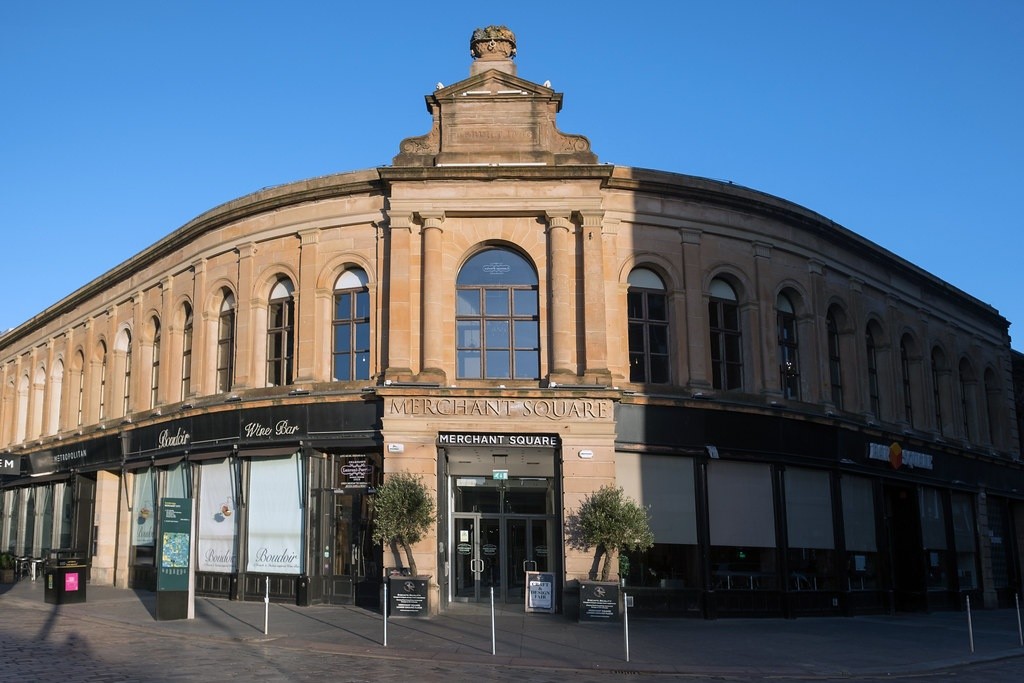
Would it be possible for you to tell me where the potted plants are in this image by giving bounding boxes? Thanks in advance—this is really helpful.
[362,471,433,620]
[0,552,15,583]
[564,488,655,626]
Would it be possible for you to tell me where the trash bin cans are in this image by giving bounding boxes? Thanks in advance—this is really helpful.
[43,548,87,605]
